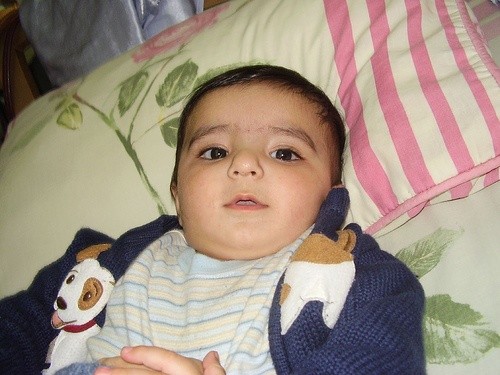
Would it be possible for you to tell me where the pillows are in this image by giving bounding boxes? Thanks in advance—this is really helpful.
[0,0,500,300]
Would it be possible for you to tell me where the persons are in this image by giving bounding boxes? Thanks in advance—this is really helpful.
[1,66,426,375]
